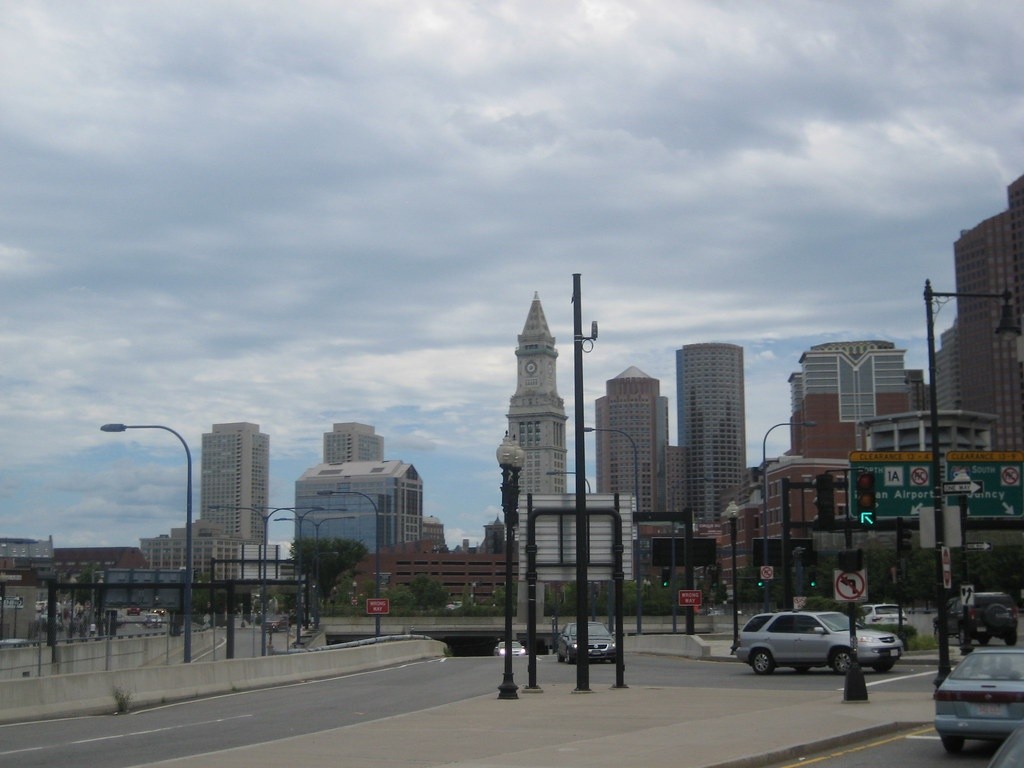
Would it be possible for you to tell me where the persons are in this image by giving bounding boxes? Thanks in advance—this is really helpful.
[89,622,96,637]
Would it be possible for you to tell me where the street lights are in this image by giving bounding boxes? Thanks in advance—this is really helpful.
[923,278,1024,689]
[673,476,712,634]
[13,596,19,638]
[100,423,192,662]
[545,427,641,633]
[497,431,525,700]
[206,488,380,656]
[763,421,817,613]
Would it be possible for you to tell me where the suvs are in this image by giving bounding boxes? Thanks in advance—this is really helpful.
[731,593,1017,675]
[262,613,290,632]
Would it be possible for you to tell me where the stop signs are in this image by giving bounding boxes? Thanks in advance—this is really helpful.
[941,547,952,589]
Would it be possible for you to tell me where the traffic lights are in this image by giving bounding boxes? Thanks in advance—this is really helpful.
[757,576,763,587]
[856,472,876,527]
[662,569,670,588]
[810,572,816,587]
[897,517,912,551]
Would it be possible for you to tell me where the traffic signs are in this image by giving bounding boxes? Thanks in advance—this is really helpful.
[849,450,1024,519]
[967,542,991,550]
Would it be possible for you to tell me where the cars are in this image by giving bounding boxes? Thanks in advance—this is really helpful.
[494,622,616,664]
[934,643,1024,768]
[126,607,166,628]
